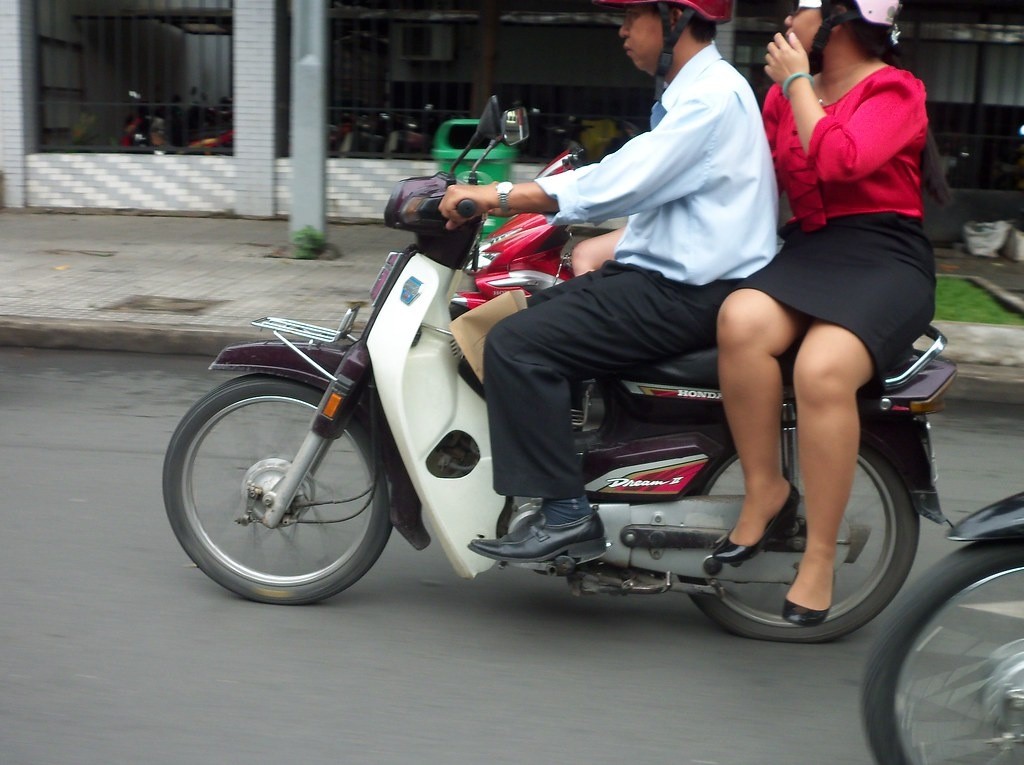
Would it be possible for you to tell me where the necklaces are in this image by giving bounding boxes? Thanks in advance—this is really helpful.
[818,95,824,105]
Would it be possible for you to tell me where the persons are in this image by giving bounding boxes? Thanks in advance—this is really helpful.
[711,0,944,626]
[439,0,783,569]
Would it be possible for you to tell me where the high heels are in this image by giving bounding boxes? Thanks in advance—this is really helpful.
[781,571,835,628]
[713,482,800,563]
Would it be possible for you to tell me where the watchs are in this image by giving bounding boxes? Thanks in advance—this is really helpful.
[497,181,514,216]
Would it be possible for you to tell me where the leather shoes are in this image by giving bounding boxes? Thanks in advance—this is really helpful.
[467,511,606,563]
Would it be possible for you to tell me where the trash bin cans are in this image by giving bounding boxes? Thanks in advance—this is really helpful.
[429,118,521,238]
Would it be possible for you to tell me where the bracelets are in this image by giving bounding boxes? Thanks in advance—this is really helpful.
[783,72,815,99]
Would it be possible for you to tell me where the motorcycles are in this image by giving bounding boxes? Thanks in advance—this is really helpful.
[860,492,1023,765]
[164,94,958,646]
[121,90,434,160]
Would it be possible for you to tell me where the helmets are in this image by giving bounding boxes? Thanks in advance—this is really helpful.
[597,0,733,25]
[787,0,900,27]
